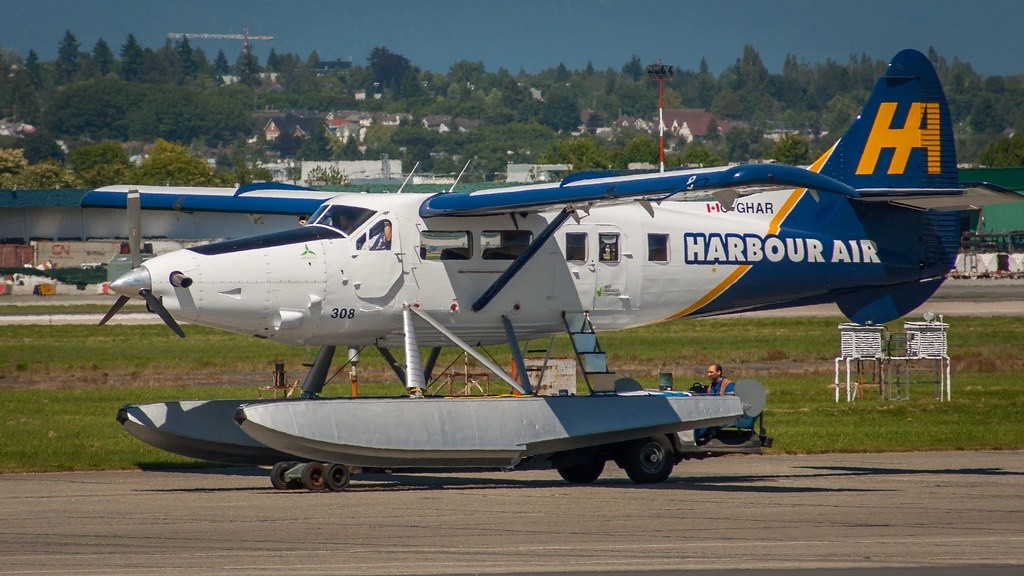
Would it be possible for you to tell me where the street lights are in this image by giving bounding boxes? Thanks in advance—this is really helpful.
[645,57,673,171]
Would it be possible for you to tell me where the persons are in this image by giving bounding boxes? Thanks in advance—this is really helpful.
[362,220,391,250]
[339,214,364,243]
[694,364,734,436]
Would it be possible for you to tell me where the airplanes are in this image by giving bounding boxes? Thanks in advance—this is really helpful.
[76,48,981,488]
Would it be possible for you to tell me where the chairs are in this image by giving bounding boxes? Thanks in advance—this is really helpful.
[418,246,585,260]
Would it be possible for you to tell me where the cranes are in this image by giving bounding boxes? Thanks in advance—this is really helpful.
[167,26,276,54]
